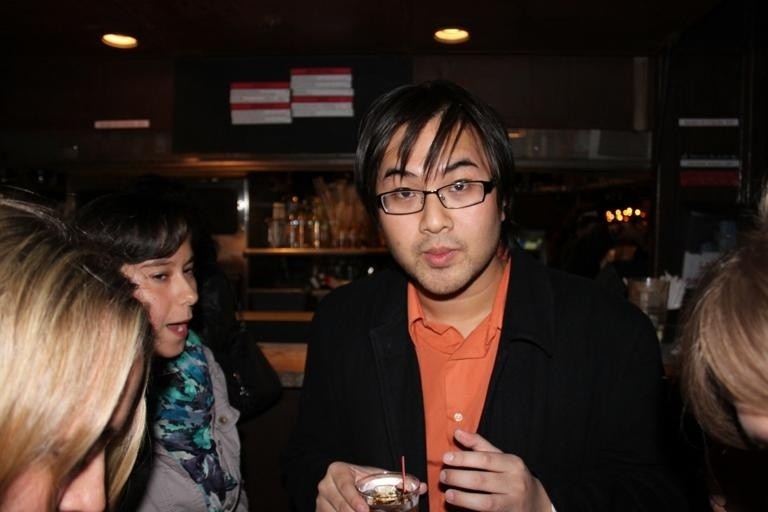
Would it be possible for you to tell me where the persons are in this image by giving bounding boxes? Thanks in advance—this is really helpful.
[169,193,283,420]
[0,183,154,511]
[271,75,717,511]
[664,233,767,511]
[64,179,255,512]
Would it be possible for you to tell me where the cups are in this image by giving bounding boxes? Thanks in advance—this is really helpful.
[355,471,422,512]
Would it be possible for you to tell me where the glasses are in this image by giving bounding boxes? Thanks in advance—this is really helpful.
[373,176,500,217]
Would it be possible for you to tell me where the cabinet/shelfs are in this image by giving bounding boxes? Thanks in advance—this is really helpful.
[83,151,668,392]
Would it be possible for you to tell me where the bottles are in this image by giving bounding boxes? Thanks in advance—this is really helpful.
[284,195,327,248]
